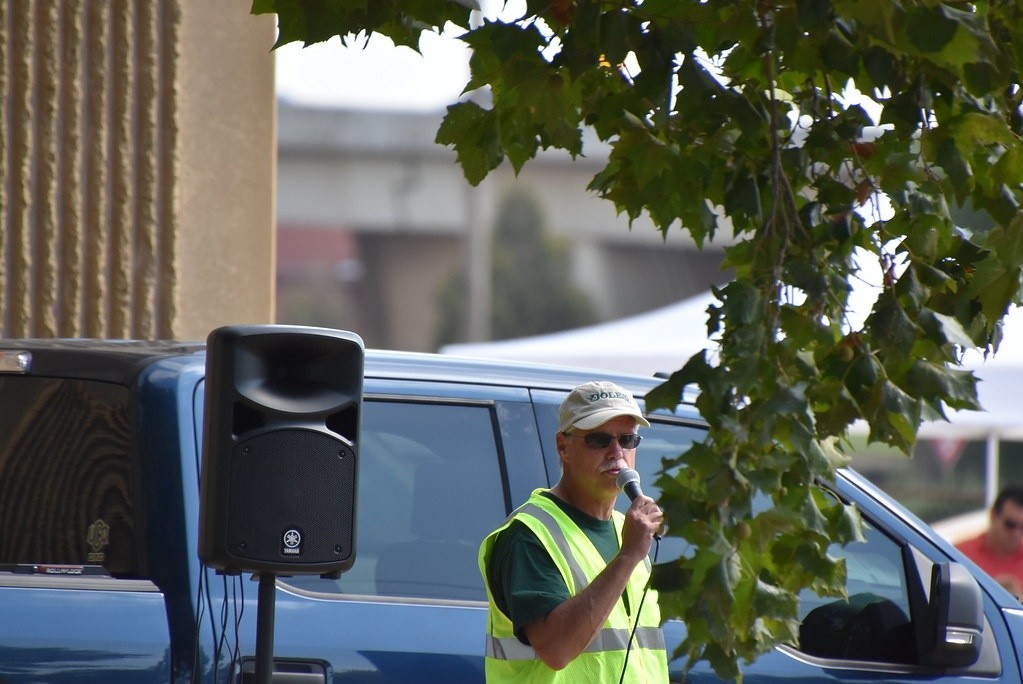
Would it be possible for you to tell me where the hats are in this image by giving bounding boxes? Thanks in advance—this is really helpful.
[555,380,651,433]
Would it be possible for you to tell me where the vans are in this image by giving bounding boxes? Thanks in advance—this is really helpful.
[0,325,1021,684]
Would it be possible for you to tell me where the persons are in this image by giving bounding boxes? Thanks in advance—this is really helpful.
[953,486,1023,602]
[477,380,671,684]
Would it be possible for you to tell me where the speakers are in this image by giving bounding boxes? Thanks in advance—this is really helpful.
[197,324,366,580]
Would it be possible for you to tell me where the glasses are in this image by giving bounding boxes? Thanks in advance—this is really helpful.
[568,431,643,450]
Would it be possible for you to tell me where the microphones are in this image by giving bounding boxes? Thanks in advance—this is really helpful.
[615,467,662,542]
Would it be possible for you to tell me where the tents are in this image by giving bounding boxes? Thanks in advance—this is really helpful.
[438,179,1023,509]
